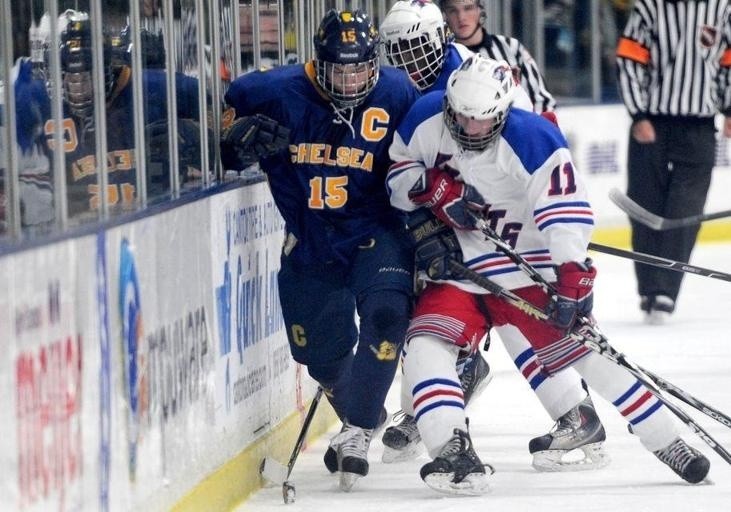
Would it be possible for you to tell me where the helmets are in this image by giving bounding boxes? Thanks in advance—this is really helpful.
[442,54,512,150]
[313,9,379,106]
[57,19,110,115]
[377,0,450,91]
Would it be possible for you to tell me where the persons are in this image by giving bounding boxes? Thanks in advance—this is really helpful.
[383,53,718,494]
[219,7,429,493]
[454,0,558,122]
[616,0,731,312]
[378,0,608,473]
[2,0,299,253]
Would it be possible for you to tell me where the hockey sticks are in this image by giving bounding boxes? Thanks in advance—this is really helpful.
[609,188,730,231]
[283,384,324,504]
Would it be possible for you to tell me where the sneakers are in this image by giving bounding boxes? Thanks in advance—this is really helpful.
[324,406,420,477]
[419,430,484,482]
[654,437,710,484]
[529,395,605,453]
[454,348,489,409]
[640,295,675,313]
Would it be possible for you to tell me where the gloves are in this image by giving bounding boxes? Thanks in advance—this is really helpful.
[146,117,216,173]
[224,114,291,170]
[543,258,596,329]
[403,168,484,279]
